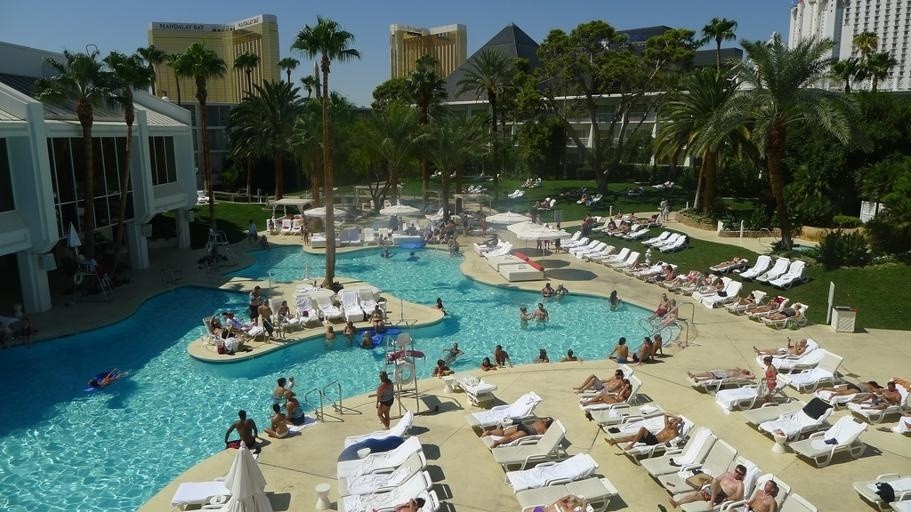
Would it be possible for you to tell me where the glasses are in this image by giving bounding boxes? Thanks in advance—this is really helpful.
[736,470,744,475]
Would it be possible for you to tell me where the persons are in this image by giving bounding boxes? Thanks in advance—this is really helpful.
[264,376,304,439]
[205,279,383,347]
[0,317,35,346]
[520,283,568,329]
[465,185,470,195]
[668,464,747,508]
[429,207,468,256]
[482,416,551,448]
[648,256,802,329]
[604,416,683,453]
[609,290,621,310]
[577,189,669,238]
[728,480,778,512]
[433,343,577,380]
[383,246,388,258]
[249,219,274,248]
[571,334,663,406]
[528,493,587,512]
[816,380,910,415]
[375,370,394,431]
[91,367,130,389]
[373,498,424,511]
[686,335,808,395]
[534,199,550,207]
[225,410,258,449]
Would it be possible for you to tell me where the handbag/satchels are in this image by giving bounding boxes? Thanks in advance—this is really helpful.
[718,291,727,296]
[686,473,713,490]
[876,482,894,501]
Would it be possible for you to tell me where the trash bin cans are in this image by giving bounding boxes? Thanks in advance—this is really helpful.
[831,306,857,333]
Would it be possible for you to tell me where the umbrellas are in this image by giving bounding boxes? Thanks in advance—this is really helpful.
[379,202,420,229]
[518,227,571,272]
[225,441,272,511]
[303,205,347,237]
[507,221,545,256]
[67,221,84,273]
[485,211,532,243]
